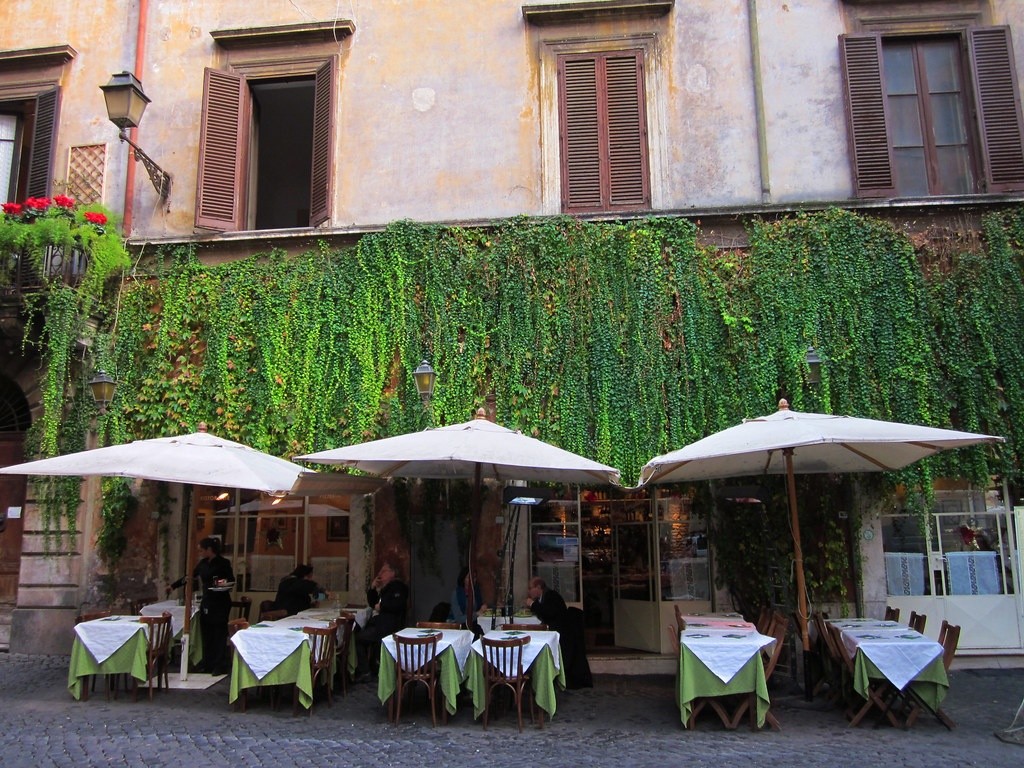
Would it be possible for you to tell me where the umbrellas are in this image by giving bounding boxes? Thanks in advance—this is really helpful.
[638,397,1007,704]
[0,421,319,682]
[290,407,623,633]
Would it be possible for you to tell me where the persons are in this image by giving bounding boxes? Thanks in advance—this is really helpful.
[524,577,593,696]
[162,538,235,677]
[446,566,496,642]
[353,559,409,684]
[268,563,337,616]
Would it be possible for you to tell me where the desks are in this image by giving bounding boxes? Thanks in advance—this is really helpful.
[67,615,158,702]
[464,629,567,730]
[840,627,958,732]
[675,629,778,730]
[476,612,543,638]
[225,621,331,716]
[825,617,902,634]
[682,612,756,631]
[299,608,371,686]
[140,599,203,667]
[377,626,475,716]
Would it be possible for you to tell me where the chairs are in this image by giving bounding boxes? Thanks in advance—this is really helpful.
[74,602,962,736]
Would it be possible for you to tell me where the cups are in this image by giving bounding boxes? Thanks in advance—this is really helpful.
[483,609,493,616]
[908,627,914,636]
[496,609,502,616]
[212,576,218,588]
[524,608,531,616]
[880,620,885,627]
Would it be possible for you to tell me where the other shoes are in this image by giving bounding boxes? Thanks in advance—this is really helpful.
[192,659,212,672]
[213,666,226,676]
[354,672,371,685]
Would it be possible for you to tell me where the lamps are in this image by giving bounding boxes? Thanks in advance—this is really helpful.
[100,71,174,199]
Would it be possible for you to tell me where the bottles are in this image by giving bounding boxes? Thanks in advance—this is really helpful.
[531,483,707,575]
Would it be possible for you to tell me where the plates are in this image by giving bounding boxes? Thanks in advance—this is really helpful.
[208,581,236,592]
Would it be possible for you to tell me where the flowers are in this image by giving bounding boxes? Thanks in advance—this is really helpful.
[0,195,108,233]
